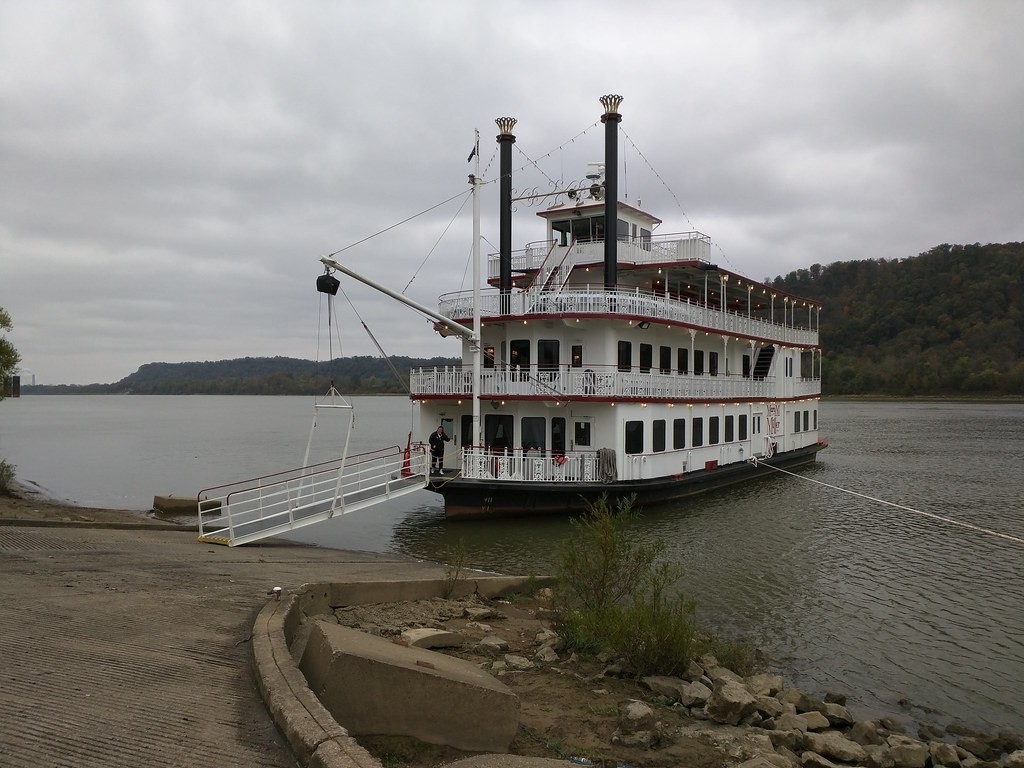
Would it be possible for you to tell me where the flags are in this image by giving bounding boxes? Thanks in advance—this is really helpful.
[467,140,479,162]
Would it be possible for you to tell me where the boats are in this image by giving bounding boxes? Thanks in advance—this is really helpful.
[317,94,831,523]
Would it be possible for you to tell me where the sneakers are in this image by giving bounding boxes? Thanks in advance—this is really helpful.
[439,469,444,474]
[431,467,435,473]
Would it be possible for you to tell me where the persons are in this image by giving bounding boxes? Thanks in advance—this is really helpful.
[429,425,450,475]
